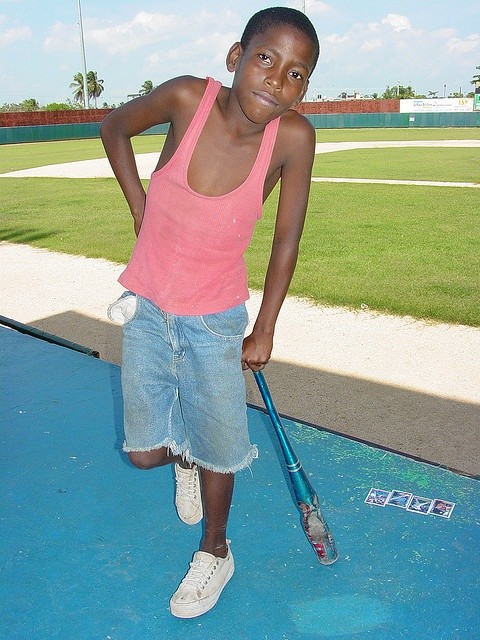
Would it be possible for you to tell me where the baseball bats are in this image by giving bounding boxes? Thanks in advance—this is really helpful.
[252,370,339,565]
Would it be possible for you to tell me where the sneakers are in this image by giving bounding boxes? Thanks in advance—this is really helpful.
[170,539,235,619]
[174,462,204,526]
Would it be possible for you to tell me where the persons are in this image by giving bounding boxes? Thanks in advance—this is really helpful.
[100,7,320,619]
